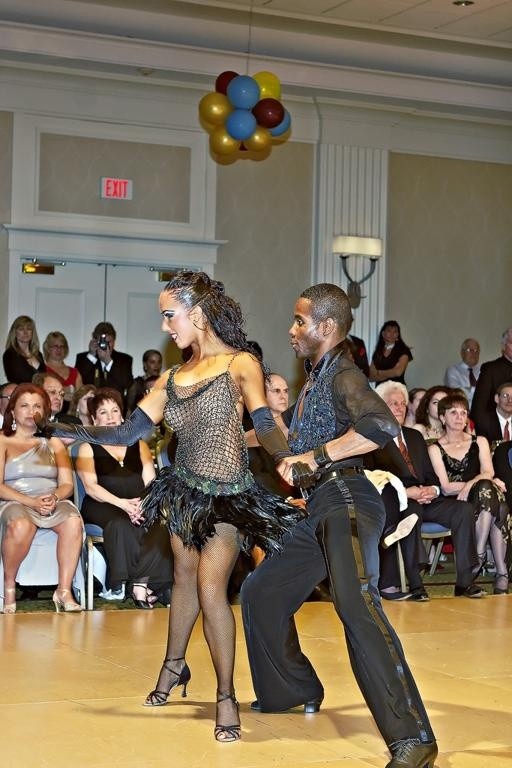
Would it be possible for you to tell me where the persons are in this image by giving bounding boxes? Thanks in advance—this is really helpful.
[0,316,173,616]
[33,266,318,742]
[360,319,512,602]
[238,281,440,766]
[239,374,307,510]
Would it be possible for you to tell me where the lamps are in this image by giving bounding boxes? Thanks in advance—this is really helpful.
[329,232,385,309]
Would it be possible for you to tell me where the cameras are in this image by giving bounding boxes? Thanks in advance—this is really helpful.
[97,334,110,351]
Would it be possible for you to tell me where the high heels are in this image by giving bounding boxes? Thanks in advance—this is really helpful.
[144,657,191,707]
[2,588,17,614]
[494,570,509,595]
[53,588,82,612]
[120,581,151,609]
[214,689,241,743]
[472,551,487,581]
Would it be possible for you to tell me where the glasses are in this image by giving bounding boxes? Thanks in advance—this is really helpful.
[465,348,478,352]
[503,393,508,399]
[47,389,65,397]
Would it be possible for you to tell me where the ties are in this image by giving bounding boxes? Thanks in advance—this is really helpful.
[398,434,414,478]
[469,368,476,386]
[504,422,509,441]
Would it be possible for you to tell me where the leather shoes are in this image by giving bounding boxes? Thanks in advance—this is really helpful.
[385,738,438,768]
[380,513,420,550]
[250,688,324,712]
[379,584,487,600]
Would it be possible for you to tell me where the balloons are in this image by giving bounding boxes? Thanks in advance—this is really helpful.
[194,65,297,167]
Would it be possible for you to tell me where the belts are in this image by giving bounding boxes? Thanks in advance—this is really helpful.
[315,466,365,488]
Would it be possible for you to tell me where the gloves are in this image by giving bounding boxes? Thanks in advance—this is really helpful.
[33,405,154,446]
[249,407,320,489]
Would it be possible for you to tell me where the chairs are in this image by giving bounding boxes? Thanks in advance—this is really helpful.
[1,436,81,612]
[71,442,109,616]
[419,516,455,578]
